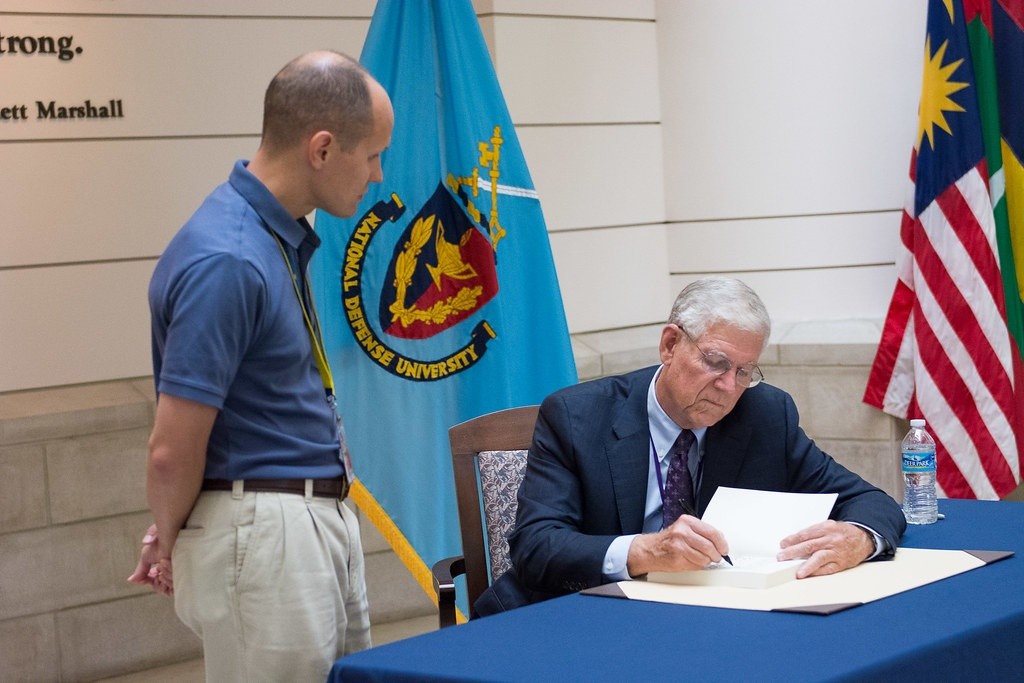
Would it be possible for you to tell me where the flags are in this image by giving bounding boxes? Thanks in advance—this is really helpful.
[860,0,1023,501]
[308,0,579,624]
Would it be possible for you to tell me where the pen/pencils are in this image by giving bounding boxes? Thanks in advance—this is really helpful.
[678,498,732,565]
[905,514,945,520]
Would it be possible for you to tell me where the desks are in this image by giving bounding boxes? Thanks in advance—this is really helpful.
[326,500,1024,683]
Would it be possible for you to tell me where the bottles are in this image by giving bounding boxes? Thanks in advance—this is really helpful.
[900,419,939,525]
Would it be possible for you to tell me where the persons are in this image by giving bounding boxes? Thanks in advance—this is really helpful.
[464,276,907,624]
[125,50,396,683]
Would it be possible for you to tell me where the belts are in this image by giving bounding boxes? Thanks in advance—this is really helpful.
[201,475,351,502]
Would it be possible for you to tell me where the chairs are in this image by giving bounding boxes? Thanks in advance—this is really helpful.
[432,404,542,627]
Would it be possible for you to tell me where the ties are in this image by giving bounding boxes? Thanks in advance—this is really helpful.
[663,429,698,531]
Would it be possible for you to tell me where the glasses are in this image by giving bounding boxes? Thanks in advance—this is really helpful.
[678,324,764,389]
